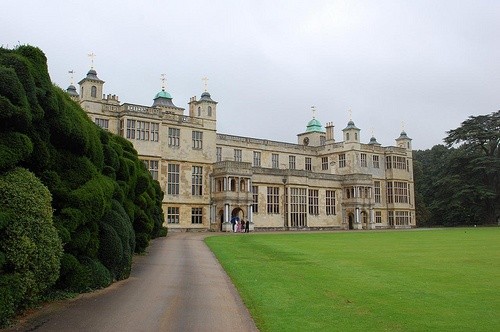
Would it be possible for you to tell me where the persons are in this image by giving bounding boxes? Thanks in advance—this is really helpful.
[245,219,250,233]
[240,219,245,233]
[230,217,241,233]
